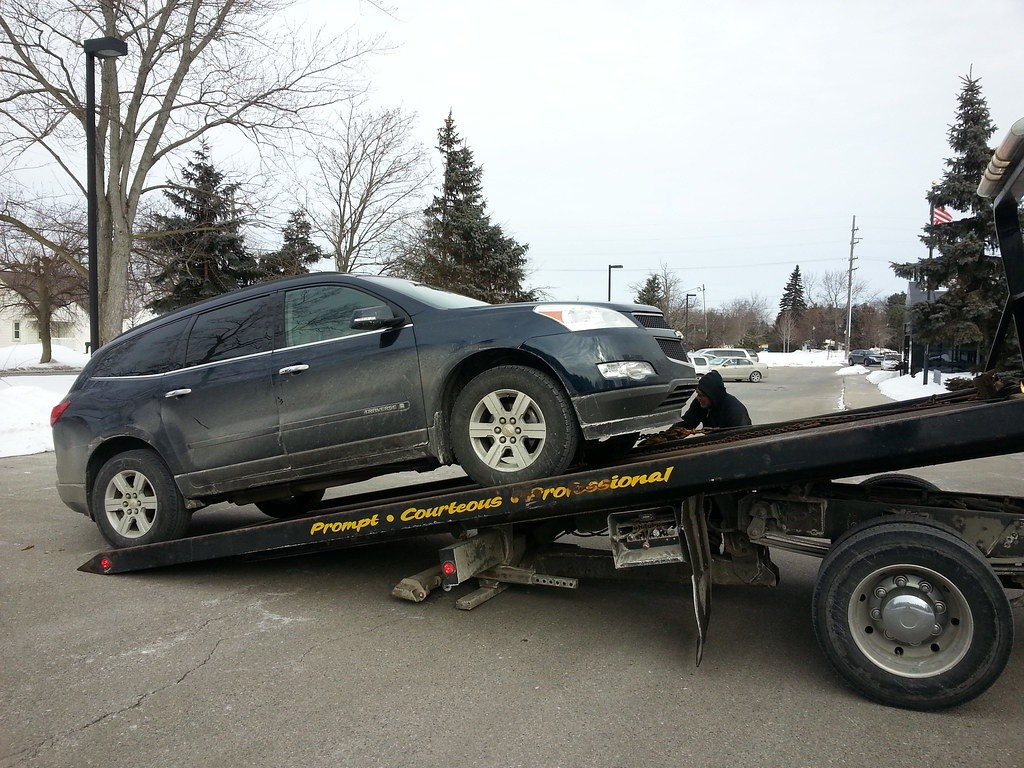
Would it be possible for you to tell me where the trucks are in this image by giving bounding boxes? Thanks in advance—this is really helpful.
[77,118,1024,713]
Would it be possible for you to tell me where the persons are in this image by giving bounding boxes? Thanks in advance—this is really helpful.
[665,370,752,561]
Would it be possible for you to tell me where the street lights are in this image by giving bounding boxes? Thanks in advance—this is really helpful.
[82,36,129,353]
[607,264,622,302]
[686,294,697,353]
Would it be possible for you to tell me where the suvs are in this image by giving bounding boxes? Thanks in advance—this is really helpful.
[848,350,884,367]
[49,270,702,547]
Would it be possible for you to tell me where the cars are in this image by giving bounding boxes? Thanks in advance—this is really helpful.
[709,356,769,384]
[878,351,899,357]
[880,353,902,371]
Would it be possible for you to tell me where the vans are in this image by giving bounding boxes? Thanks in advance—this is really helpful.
[694,348,760,362]
[687,355,710,381]
